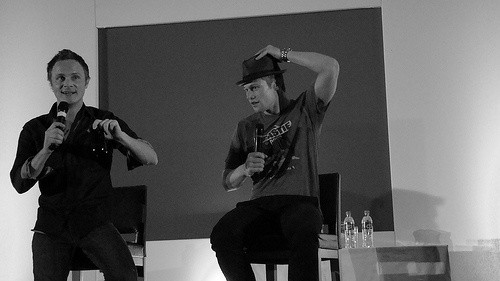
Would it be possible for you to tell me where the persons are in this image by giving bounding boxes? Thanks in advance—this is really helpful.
[10,49,158,280]
[209,45,339,281]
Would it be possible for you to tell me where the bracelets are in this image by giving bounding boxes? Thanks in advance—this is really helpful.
[279,47,292,62]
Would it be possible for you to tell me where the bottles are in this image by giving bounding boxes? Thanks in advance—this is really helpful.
[361,210,373,248]
[344,210,355,248]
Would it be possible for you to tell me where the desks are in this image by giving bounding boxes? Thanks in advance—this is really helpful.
[336,245,452,281]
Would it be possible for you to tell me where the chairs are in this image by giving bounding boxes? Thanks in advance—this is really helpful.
[69,185,148,281]
[245,173,344,281]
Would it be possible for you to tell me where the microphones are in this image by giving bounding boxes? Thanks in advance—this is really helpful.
[54,100,70,146]
[254,121,266,158]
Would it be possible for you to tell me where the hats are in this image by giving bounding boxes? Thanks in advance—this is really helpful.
[236,53,287,87]
